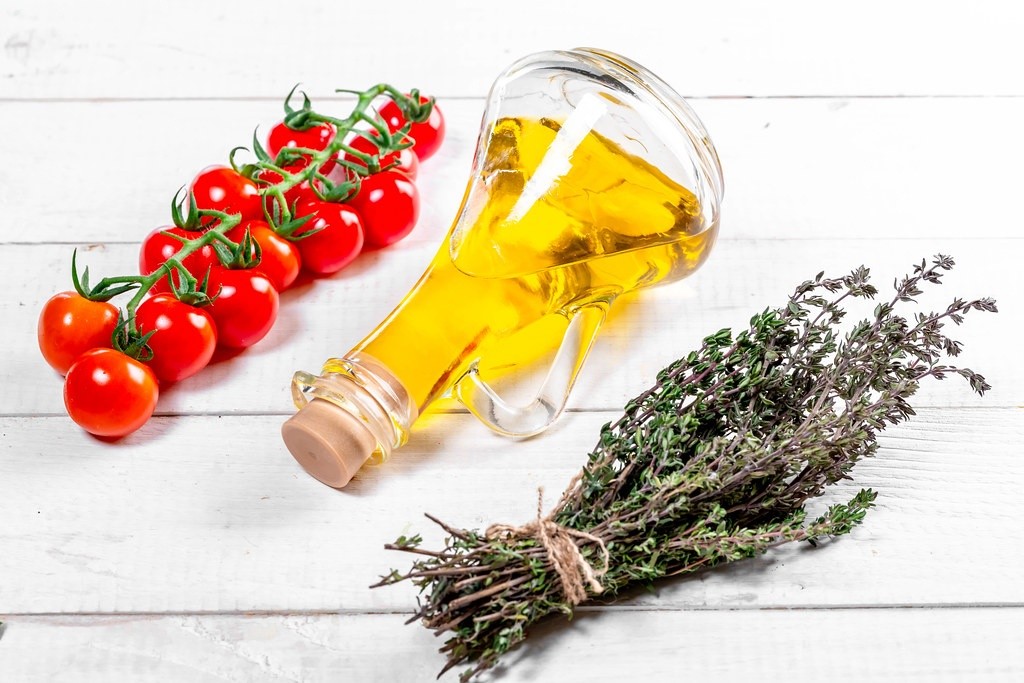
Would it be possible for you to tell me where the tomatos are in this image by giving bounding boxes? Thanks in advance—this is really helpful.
[38,83,445,439]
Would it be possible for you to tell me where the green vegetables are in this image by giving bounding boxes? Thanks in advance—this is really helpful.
[366,252,999,683]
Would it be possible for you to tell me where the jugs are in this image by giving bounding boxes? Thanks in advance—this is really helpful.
[281,47,725,489]
